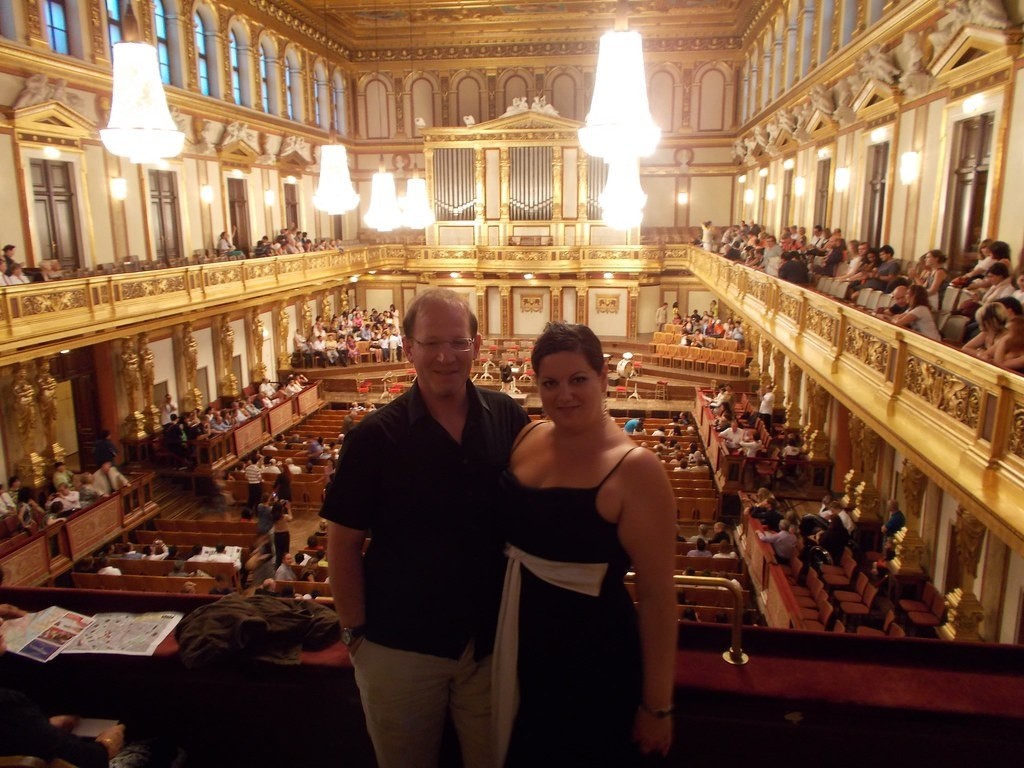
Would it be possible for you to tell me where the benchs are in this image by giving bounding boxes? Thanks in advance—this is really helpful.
[40,404,761,624]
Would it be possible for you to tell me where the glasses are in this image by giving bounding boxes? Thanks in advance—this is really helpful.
[406,334,477,353]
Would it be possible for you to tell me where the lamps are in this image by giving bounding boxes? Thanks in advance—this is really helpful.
[311,0,360,216]
[362,0,403,232]
[98,0,186,161]
[402,0,436,229]
[576,0,661,158]
[599,158,647,231]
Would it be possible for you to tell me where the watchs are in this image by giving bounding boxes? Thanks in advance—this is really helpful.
[340,626,365,647]
[641,700,673,719]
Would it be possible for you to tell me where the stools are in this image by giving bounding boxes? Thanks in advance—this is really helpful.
[655,381,669,401]
[479,345,537,385]
[354,367,417,403]
[615,387,627,402]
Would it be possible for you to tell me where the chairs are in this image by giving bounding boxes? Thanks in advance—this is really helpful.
[795,576,823,608]
[803,600,834,630]
[833,572,869,602]
[857,623,905,638]
[799,589,829,619]
[823,554,857,585]
[288,305,410,368]
[783,557,804,575]
[840,582,879,614]
[819,546,853,573]
[834,620,846,633]
[632,362,640,377]
[791,567,818,596]
[642,224,980,378]
[884,609,895,632]
[908,598,944,625]
[698,384,805,491]
[1,238,369,281]
[898,582,936,612]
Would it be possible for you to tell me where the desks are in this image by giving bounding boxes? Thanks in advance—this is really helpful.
[800,455,834,494]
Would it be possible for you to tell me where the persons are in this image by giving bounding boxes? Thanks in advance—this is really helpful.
[294,305,403,369]
[77,541,233,595]
[34,259,64,282]
[0,429,133,531]
[0,566,125,768]
[256,491,277,537]
[623,412,710,472]
[675,509,754,625]
[272,498,293,570]
[341,411,357,435]
[656,300,744,348]
[161,373,307,467]
[501,320,677,768]
[218,223,246,257]
[254,222,345,258]
[254,518,329,601]
[499,360,513,391]
[0,244,30,287]
[707,383,906,590]
[318,288,532,768]
[228,432,344,513]
[350,402,377,412]
[699,220,1024,367]
